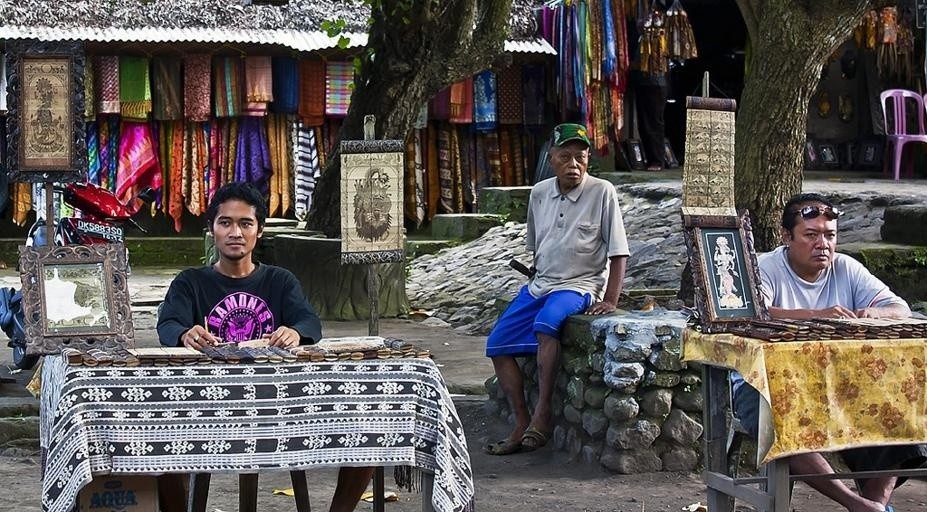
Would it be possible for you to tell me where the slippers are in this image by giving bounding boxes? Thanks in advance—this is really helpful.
[521,426,553,452]
[487,438,522,456]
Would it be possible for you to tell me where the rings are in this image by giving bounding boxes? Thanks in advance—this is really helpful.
[193,334,199,343]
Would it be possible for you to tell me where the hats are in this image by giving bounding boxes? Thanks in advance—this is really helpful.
[553,122,591,146]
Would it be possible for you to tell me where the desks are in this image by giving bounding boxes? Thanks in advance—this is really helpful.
[40,348,475,512]
[681,322,927,512]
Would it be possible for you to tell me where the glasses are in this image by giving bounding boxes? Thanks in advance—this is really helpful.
[791,206,841,219]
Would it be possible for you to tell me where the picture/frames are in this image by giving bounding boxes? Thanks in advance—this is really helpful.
[683,216,772,321]
[4,42,89,183]
[18,241,132,353]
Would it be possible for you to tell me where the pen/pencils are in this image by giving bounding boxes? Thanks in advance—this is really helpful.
[204,316,208,333]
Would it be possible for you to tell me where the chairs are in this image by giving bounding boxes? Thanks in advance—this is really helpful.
[880,88,927,180]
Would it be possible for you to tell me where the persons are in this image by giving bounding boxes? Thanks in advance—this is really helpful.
[633,7,674,172]
[730,191,927,512]
[158,182,376,512]
[483,120,631,455]
